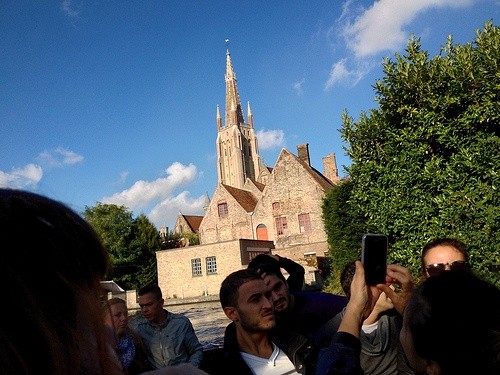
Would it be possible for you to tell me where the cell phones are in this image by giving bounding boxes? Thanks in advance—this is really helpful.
[362,234,388,285]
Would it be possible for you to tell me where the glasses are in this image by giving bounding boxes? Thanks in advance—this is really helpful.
[424,261,471,277]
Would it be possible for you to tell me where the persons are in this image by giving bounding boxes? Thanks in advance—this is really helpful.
[108,238,500,375]
[0,188,125,375]
